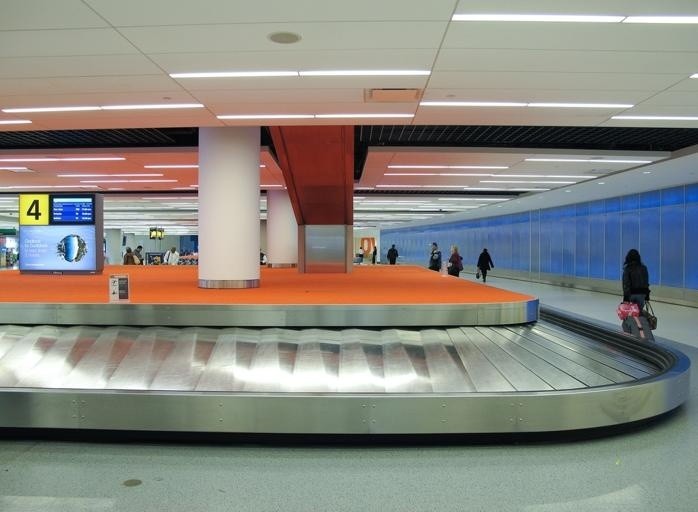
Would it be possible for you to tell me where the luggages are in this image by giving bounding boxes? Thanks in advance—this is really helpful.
[623,316,654,341]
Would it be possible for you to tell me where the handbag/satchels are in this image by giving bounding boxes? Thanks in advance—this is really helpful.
[642,310,656,330]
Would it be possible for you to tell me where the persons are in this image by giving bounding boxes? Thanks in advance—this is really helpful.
[122,246,138,265]
[358,246,364,263]
[386,244,399,265]
[621,248,651,333]
[260,248,268,265]
[163,247,180,266]
[132,246,144,265]
[446,243,464,277]
[372,246,377,264]
[428,242,442,272]
[476,248,495,283]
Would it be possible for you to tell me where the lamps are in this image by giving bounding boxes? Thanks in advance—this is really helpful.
[150,226,164,239]
